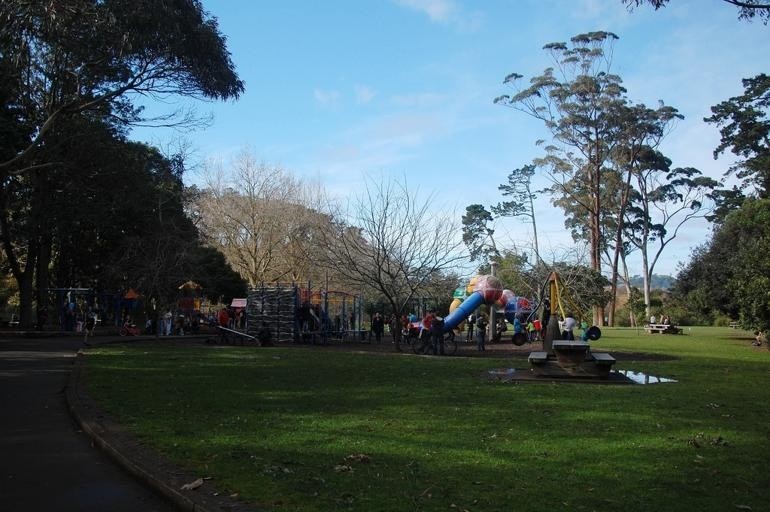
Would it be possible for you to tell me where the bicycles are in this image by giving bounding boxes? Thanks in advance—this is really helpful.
[119,321,141,337]
[412,330,458,357]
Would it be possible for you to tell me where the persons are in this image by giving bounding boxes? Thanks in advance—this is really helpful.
[63,295,96,344]
[514,313,541,344]
[649,312,671,325]
[205,305,247,345]
[561,313,588,340]
[123,309,201,334]
[463,310,507,352]
[369,309,445,356]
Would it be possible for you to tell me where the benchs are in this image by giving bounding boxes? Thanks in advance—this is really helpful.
[644,327,665,333]
[730,322,741,329]
[591,352,616,376]
[528,350,548,375]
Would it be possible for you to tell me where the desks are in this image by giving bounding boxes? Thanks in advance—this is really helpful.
[343,329,371,345]
[552,339,590,373]
[650,324,670,329]
